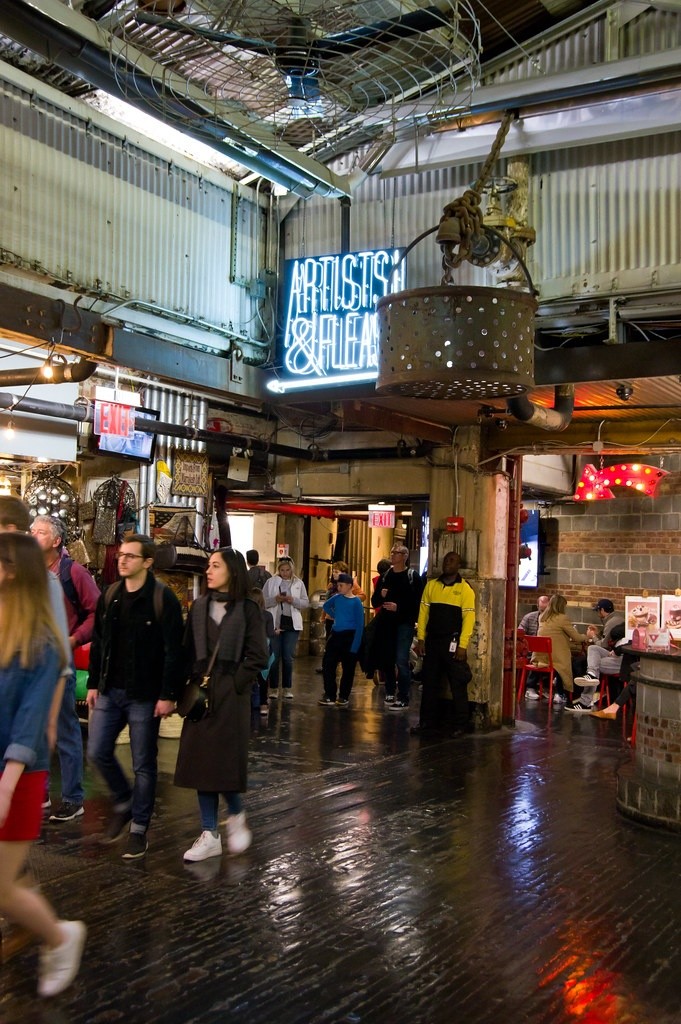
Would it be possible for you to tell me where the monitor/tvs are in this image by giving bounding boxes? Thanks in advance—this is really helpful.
[93,407,160,464]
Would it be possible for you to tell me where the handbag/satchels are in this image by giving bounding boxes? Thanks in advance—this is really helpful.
[177,680,213,722]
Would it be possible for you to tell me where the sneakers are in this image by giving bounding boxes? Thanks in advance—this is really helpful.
[98,805,135,844]
[228,810,251,854]
[249,661,475,745]
[522,670,602,712]
[49,802,85,822]
[42,792,51,808]
[37,920,88,997]
[122,832,149,858]
[183,830,223,862]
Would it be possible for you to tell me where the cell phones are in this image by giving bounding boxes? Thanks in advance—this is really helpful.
[280,592,287,597]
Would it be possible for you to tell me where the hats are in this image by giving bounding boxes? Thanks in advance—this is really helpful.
[591,599,614,612]
[336,573,353,585]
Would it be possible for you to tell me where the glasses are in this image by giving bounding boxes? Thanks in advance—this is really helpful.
[203,546,237,560]
[390,551,406,555]
[278,557,293,564]
[114,551,147,561]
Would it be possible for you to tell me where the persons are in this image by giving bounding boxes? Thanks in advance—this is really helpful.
[0,530,87,998]
[242,538,637,740]
[0,495,30,535]
[25,514,100,822]
[81,533,188,859]
[171,545,278,863]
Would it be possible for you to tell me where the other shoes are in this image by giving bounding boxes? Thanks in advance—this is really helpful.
[588,710,617,721]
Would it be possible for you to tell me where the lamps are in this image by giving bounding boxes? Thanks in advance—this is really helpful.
[42,354,68,378]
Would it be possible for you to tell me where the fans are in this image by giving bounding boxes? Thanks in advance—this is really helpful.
[105,0,482,154]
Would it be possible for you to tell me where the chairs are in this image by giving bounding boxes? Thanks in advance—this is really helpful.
[598,673,633,715]
[517,635,574,710]
